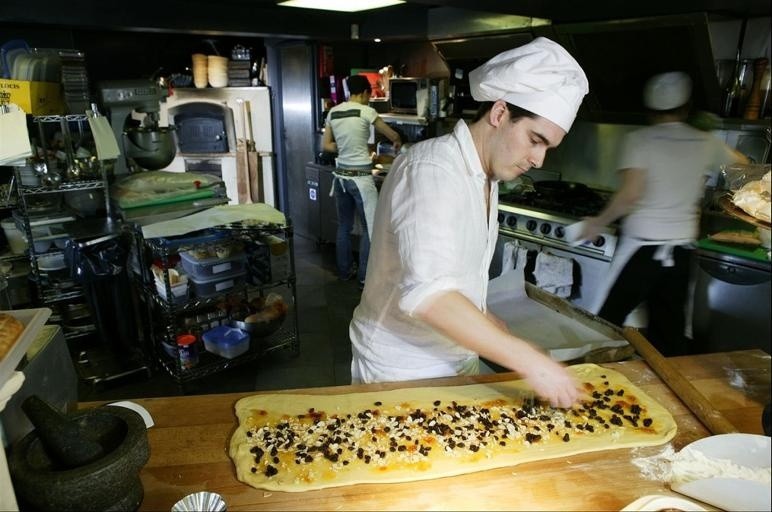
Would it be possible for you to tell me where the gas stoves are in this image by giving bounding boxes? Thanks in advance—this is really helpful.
[497,188,624,261]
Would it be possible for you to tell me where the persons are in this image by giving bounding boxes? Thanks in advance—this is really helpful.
[347,37,591,410]
[320,76,404,290]
[569,72,750,357]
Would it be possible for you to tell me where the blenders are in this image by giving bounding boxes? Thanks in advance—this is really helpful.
[95,76,179,176]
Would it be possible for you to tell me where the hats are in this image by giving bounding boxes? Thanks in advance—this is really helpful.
[468,37,591,136]
[643,66,695,111]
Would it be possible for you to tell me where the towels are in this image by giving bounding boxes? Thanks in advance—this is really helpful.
[499,241,529,278]
[534,252,573,301]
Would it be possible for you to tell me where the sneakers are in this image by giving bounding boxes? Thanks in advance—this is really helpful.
[339,264,357,281]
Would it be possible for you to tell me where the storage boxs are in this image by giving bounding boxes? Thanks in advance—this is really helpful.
[154,229,289,304]
[201,326,250,359]
[0,77,67,115]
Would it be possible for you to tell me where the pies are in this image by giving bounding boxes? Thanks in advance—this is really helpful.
[228,362,680,493]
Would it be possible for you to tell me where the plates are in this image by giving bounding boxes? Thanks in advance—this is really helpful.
[30,252,67,271]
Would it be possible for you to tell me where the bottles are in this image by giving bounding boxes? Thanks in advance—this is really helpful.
[721,60,742,120]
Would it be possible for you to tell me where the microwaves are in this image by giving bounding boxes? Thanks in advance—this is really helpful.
[389,77,430,114]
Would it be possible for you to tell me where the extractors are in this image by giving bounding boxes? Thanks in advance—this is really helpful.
[428,12,722,126]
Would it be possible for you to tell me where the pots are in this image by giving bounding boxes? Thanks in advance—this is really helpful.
[532,180,589,200]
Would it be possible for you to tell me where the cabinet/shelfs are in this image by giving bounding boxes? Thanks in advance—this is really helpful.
[315,48,431,165]
[137,235,298,383]
[14,104,122,381]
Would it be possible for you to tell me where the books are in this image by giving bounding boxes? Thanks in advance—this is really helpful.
[0,110,34,168]
[89,115,121,161]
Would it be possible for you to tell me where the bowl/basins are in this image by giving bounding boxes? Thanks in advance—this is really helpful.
[231,314,288,338]
